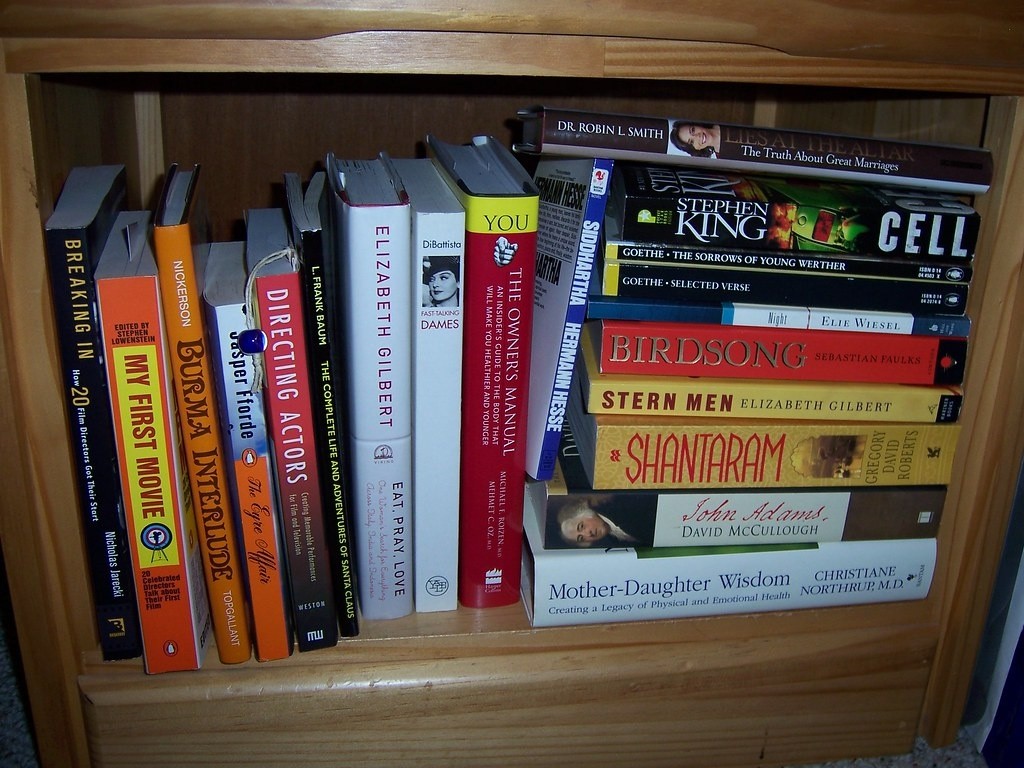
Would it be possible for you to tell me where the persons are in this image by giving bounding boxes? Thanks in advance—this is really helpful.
[421,256,459,309]
[671,121,722,159]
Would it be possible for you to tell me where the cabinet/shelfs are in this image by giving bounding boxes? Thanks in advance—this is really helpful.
[0,0,1024,768]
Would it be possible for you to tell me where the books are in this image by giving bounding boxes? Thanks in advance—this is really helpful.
[41,102,997,677]
